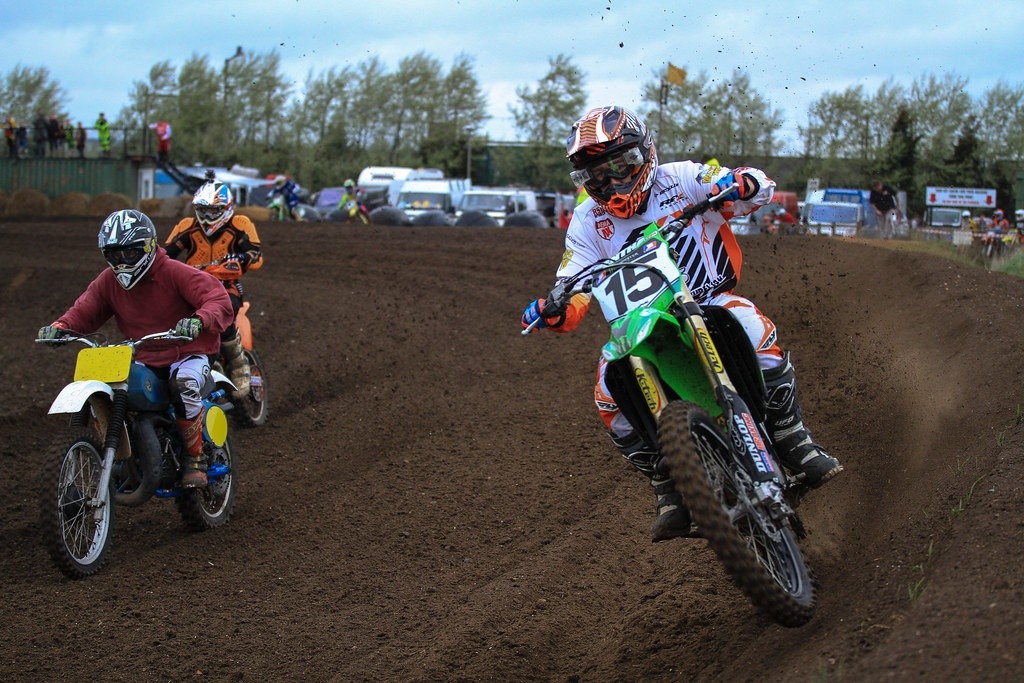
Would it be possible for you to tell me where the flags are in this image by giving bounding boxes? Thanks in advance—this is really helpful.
[666,62,687,87]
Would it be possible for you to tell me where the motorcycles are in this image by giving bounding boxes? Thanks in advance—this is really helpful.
[268,190,307,226]
[518,183,829,629]
[174,252,271,428]
[34,323,237,583]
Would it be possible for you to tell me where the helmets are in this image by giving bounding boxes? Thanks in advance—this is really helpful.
[962,210,970,220]
[994,209,1004,223]
[566,106,657,220]
[344,179,357,195]
[273,174,288,191]
[190,178,234,237]
[97,211,158,290]
[1015,209,1024,221]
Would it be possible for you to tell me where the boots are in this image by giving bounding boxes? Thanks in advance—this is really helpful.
[605,426,690,540]
[174,406,208,489]
[760,351,842,488]
[219,327,250,398]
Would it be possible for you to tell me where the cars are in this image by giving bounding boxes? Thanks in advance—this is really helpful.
[727,185,1023,261]
[304,166,576,228]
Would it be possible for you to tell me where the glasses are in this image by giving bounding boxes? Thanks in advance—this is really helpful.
[194,203,223,220]
[101,247,147,267]
[584,147,645,189]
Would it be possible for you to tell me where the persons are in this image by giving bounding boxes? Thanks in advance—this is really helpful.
[37,209,234,489]
[96,113,111,158]
[33,108,49,159]
[264,176,301,220]
[521,105,844,541]
[3,116,29,158]
[76,121,86,159]
[870,179,901,240]
[49,113,73,158]
[962,209,1024,260]
[166,179,264,401]
[149,119,173,162]
[338,179,371,225]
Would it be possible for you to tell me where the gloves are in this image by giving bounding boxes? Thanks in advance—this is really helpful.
[709,170,749,210]
[175,316,202,343]
[519,298,560,333]
[38,326,69,348]
